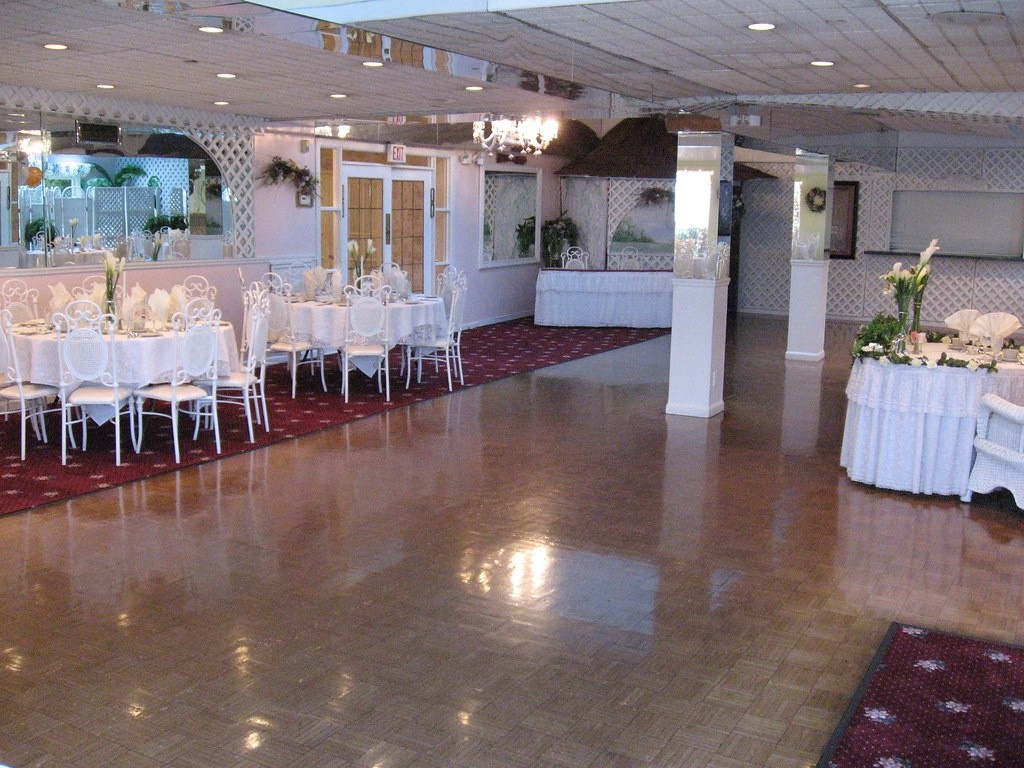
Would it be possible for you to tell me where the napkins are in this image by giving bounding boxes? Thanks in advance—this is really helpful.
[969,312,1021,338]
[943,309,983,329]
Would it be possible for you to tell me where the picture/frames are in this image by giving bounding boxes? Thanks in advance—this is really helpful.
[829,180,859,259]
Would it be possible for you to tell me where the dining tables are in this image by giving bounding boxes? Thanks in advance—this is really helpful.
[279,292,445,384]
[8,317,239,432]
[22,246,107,266]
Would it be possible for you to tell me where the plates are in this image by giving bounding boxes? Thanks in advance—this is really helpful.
[10,318,218,337]
[1003,358,1018,361]
[948,345,962,350]
[280,292,438,306]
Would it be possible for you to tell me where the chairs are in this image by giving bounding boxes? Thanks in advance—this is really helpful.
[17,227,190,267]
[558,248,589,269]
[957,394,1024,504]
[0,260,467,463]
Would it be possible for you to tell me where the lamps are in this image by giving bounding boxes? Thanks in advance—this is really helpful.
[472,113,559,161]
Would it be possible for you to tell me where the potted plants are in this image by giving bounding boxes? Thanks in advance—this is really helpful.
[514,217,535,258]
[544,210,578,258]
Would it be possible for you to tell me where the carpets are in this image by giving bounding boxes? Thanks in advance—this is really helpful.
[0,314,677,519]
[809,623,1024,768]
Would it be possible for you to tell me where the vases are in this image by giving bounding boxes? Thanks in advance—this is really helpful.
[912,297,923,332]
[355,274,364,296]
[894,297,911,337]
[105,301,117,332]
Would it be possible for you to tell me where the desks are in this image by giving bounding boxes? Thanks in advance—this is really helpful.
[839,339,1024,494]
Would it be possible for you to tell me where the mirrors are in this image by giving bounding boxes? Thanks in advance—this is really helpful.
[0,107,239,267]
[476,163,542,271]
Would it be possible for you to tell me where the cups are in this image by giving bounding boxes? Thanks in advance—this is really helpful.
[952,338,960,346]
[1003,349,1018,359]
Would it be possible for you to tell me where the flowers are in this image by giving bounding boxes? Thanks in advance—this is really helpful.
[347,239,376,273]
[880,246,940,294]
[69,218,78,237]
[908,239,939,296]
[258,156,319,197]
[152,240,163,259]
[104,253,125,299]
